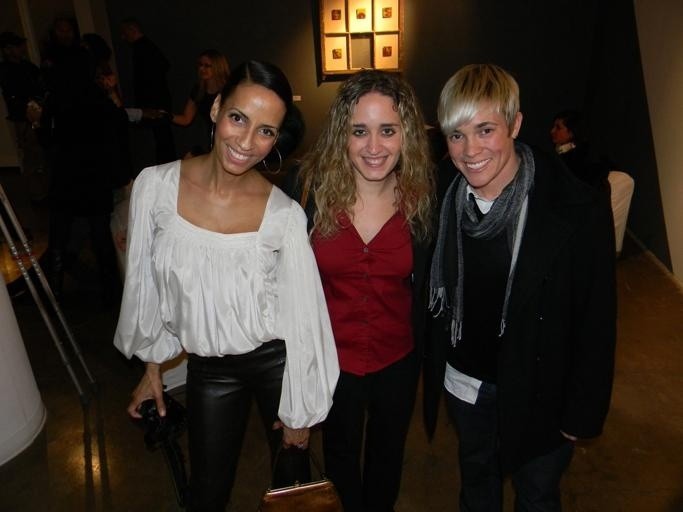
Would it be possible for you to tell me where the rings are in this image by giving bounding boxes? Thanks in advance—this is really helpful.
[296,441,305,450]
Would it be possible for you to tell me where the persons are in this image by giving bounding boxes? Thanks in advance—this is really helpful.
[295,69,439,511]
[417,64,619,512]
[0,237,53,511]
[547,104,588,189]
[113,60,341,511]
[140,88,202,161]
[158,50,234,137]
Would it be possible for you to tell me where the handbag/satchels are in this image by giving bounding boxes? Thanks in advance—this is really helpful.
[262,477,343,511]
